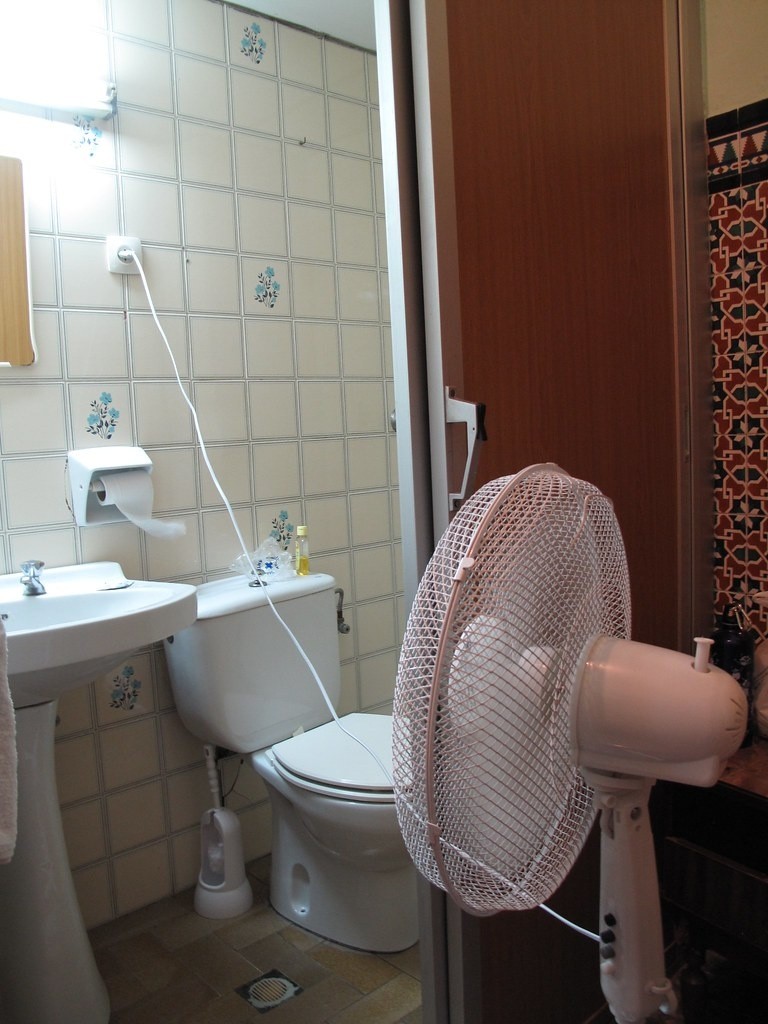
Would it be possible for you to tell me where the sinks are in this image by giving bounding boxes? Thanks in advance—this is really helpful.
[1,584,198,712]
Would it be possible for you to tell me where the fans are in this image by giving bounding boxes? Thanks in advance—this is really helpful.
[393,462,747,1024]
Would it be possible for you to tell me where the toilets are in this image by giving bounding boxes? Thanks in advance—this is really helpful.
[162,572,423,953]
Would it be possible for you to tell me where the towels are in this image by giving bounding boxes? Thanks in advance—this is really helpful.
[0,616,19,866]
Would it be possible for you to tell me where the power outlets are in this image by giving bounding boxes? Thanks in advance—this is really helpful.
[107,234,144,275]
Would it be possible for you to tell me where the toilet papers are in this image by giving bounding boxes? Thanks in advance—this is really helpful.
[94,469,187,541]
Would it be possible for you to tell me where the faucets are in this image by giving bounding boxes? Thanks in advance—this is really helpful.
[19,558,48,596]
